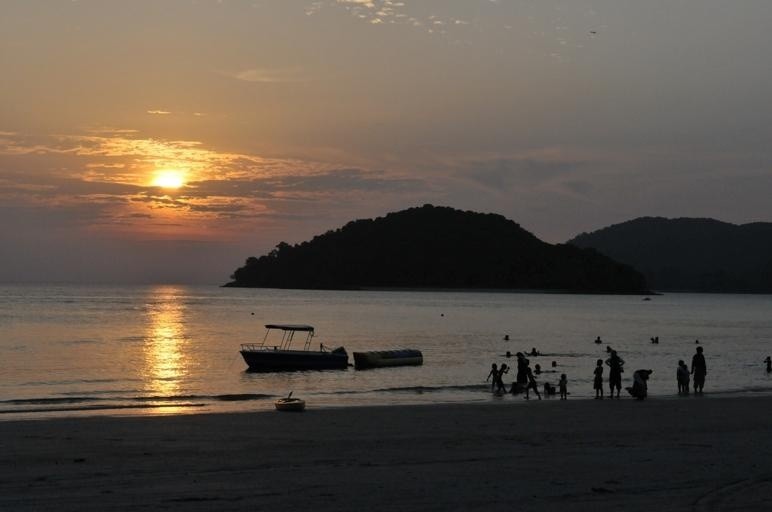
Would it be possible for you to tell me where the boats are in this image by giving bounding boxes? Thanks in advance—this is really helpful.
[237,322,350,371]
[353,349,425,369]
[273,397,306,410]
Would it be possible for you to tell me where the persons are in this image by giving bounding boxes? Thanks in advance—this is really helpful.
[485,333,708,402]
[763,356,771,372]
